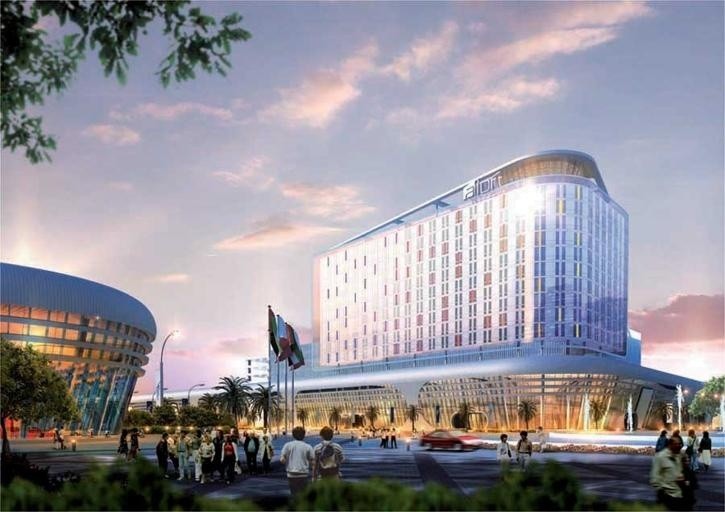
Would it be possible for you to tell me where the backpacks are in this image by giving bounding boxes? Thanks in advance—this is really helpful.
[319,442,338,471]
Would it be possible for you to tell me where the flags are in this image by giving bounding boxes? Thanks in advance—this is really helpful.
[269,307,305,371]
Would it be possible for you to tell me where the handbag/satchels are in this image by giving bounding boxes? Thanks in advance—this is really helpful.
[234,461,241,476]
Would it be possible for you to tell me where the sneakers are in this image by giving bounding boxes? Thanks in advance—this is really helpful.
[160,473,230,485]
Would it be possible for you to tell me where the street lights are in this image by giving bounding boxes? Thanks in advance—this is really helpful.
[187,382,205,403]
[156,324,184,407]
[151,388,168,401]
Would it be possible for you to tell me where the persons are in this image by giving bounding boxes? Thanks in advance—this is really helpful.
[53,426,65,450]
[515,431,532,469]
[649,428,712,511]
[117,427,397,495]
[497,433,511,462]
[537,426,546,453]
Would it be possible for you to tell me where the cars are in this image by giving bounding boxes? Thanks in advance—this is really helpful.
[420,428,482,452]
[28,426,42,438]
[135,428,144,438]
